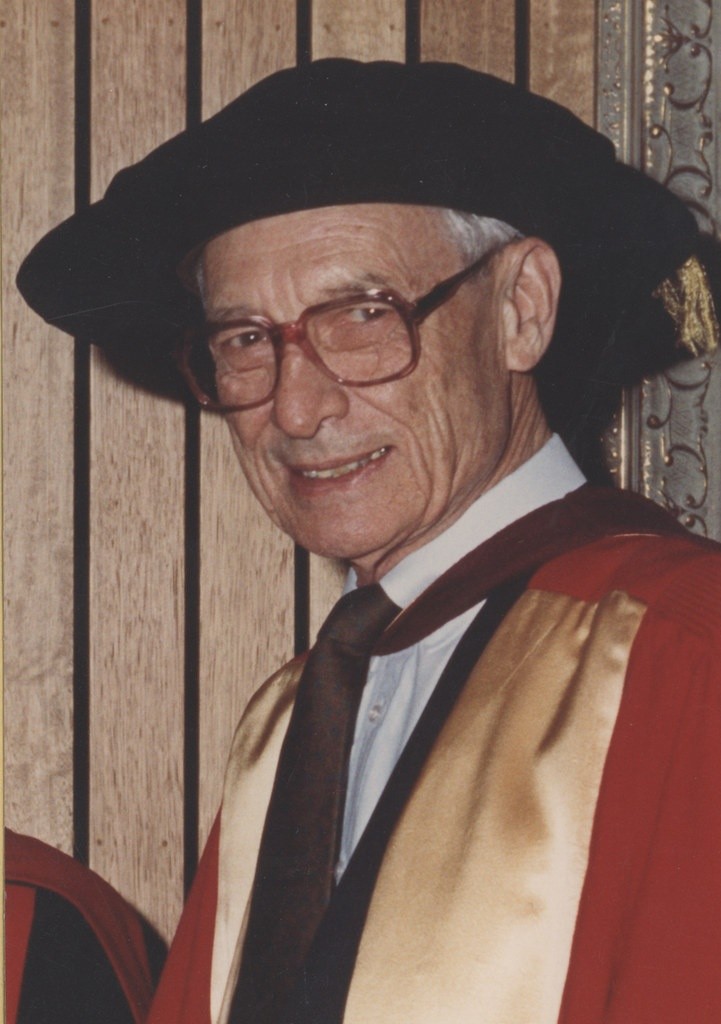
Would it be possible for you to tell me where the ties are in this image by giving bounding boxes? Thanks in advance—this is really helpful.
[227,582,403,1024]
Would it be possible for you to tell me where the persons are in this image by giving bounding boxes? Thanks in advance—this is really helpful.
[16,58,721,1024]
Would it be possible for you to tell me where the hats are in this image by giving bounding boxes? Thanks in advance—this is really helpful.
[15,56,705,402]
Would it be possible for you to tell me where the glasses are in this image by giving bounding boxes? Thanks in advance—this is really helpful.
[179,240,512,413]
